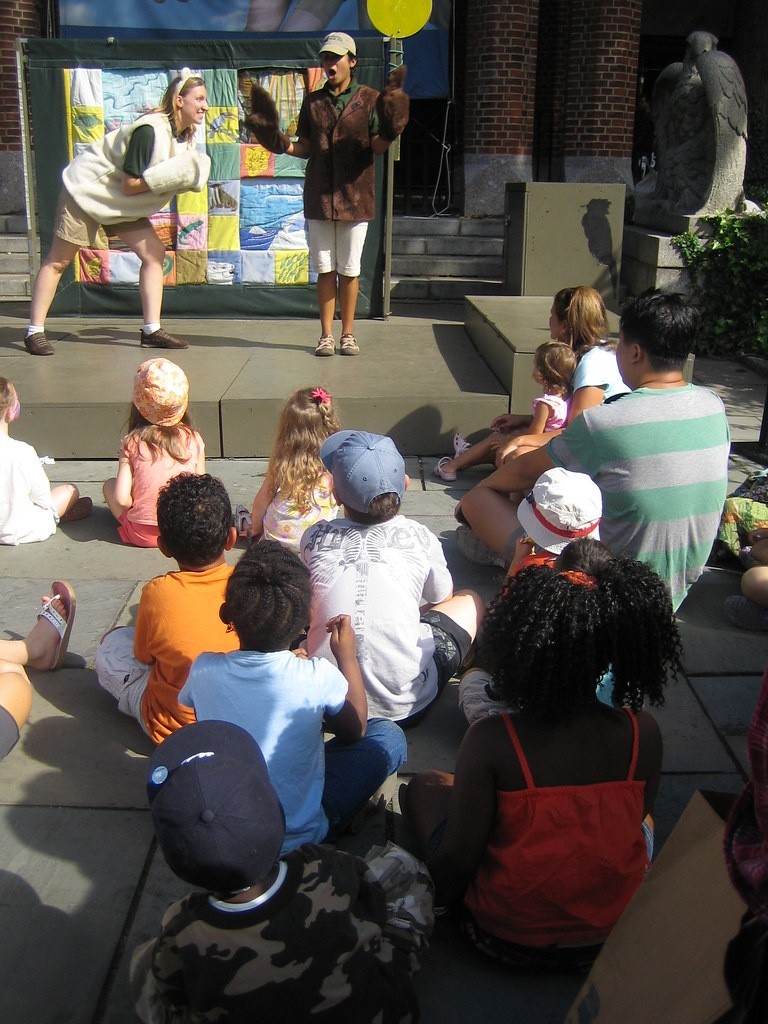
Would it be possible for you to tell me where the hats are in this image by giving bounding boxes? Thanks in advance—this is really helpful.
[517,467,602,555]
[319,32,356,55]
[133,358,188,426]
[320,430,405,513]
[146,720,286,891]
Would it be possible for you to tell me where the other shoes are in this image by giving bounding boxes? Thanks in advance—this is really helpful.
[455,526,506,568]
[740,546,763,569]
[724,594,768,631]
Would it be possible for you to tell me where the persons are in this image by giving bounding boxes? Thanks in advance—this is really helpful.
[25,67,212,357]
[301,429,484,734]
[0,378,95,545]
[123,717,436,1024]
[434,285,630,504]
[245,31,411,357]
[413,540,686,972]
[724,528,768,634]
[0,580,77,762]
[235,387,346,558]
[176,540,408,860]
[104,360,206,548]
[502,466,601,592]
[94,471,243,746]
[432,284,730,616]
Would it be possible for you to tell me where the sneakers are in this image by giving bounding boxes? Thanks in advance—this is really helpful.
[24,332,54,355]
[140,328,189,349]
[315,335,336,355]
[340,334,359,354]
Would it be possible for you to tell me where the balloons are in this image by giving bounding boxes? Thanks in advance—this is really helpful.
[365,1,434,40]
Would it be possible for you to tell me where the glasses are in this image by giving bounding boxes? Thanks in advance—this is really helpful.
[565,288,576,307]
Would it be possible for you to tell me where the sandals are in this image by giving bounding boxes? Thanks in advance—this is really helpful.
[234,504,252,533]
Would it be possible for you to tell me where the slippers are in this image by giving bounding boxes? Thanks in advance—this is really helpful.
[36,580,76,669]
[434,457,456,480]
[454,432,472,459]
